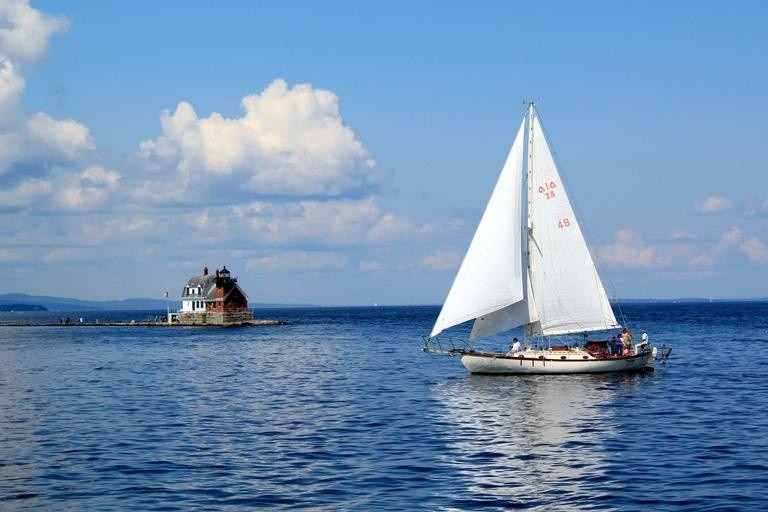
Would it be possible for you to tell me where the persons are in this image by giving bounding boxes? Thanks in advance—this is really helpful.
[609,327,649,359]
[507,338,521,356]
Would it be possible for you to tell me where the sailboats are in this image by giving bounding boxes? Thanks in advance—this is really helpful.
[420,97,659,374]
